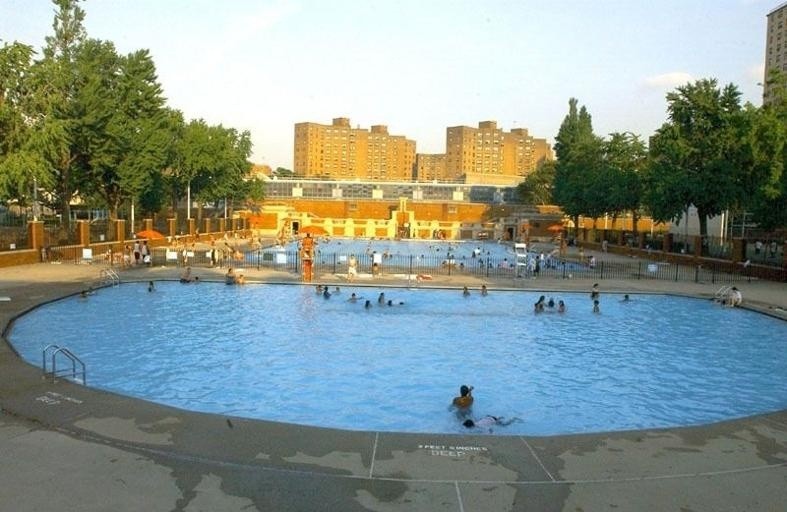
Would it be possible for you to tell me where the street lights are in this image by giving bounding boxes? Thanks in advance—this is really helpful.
[31,172,39,220]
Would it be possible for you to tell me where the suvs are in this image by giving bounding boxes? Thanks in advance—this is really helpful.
[88,216,107,232]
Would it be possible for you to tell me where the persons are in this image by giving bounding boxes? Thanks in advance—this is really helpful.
[134,228,245,292]
[720,299,732,308]
[86,287,96,295]
[459,414,525,433]
[297,235,404,308]
[446,384,475,411]
[754,238,761,256]
[769,239,777,259]
[436,240,608,314]
[78,290,86,297]
[727,285,742,309]
[617,294,633,303]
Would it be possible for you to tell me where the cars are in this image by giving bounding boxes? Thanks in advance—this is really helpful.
[645,240,689,254]
